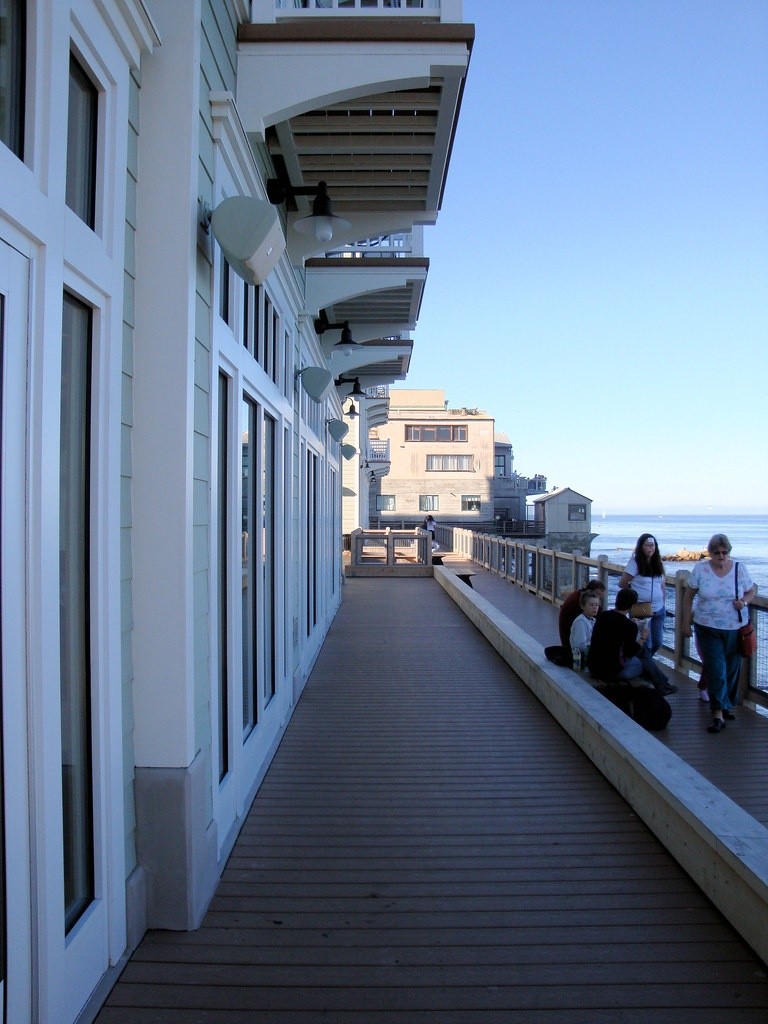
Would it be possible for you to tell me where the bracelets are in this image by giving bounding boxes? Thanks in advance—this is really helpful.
[640,637,645,640]
[740,599,747,606]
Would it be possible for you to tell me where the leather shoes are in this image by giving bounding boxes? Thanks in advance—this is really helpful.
[723,709,735,720]
[708,717,726,733]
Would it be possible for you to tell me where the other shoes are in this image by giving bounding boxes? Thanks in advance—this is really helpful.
[661,685,678,696]
[698,690,709,702]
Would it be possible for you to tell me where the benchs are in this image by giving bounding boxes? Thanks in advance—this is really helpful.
[575,658,655,718]
[449,568,477,589]
[432,553,446,565]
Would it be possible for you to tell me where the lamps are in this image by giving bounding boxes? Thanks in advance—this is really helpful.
[365,470,375,478]
[313,308,365,357]
[369,478,376,484]
[334,373,369,402]
[509,448,514,460]
[360,459,371,470]
[341,396,359,419]
[267,178,353,243]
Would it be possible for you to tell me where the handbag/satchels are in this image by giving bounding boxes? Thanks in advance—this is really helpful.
[629,576,653,618]
[738,623,756,657]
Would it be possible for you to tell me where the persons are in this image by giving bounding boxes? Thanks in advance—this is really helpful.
[423,515,440,555]
[682,534,756,734]
[697,655,709,703]
[586,588,680,697]
[558,579,598,675]
[618,532,666,659]
